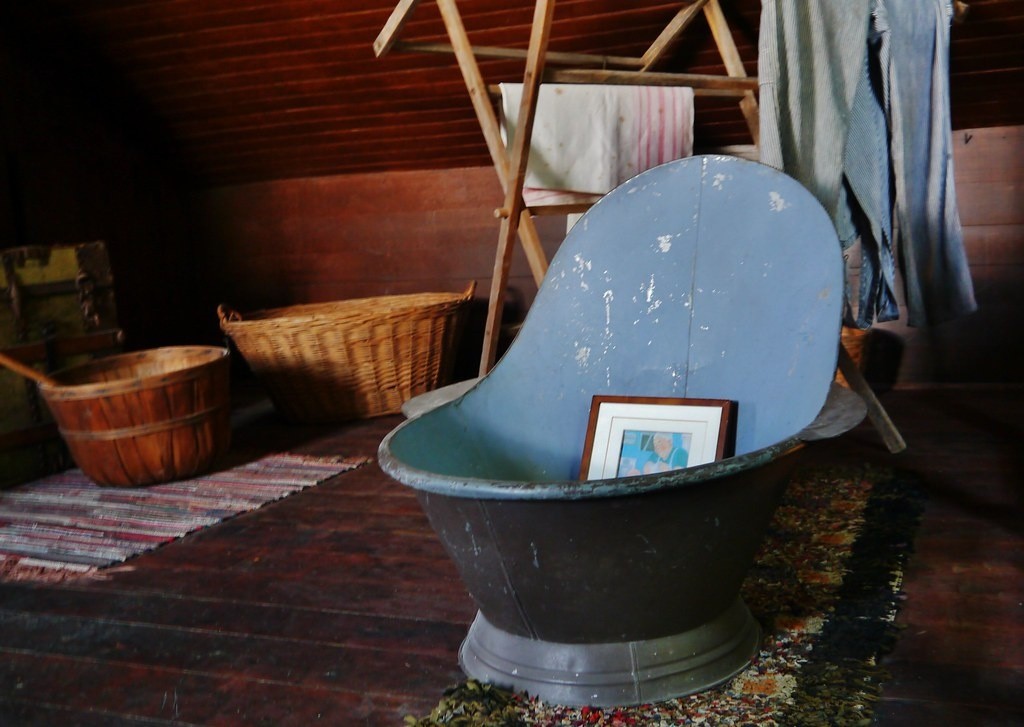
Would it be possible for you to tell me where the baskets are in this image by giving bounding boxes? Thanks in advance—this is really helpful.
[216,279,478,425]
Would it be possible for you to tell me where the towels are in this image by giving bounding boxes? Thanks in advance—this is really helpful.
[498,81,695,207]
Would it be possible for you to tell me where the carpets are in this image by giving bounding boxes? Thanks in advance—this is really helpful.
[0,440,377,583]
[404,461,928,727]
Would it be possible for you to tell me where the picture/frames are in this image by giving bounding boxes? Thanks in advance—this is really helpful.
[578,395,730,480]
